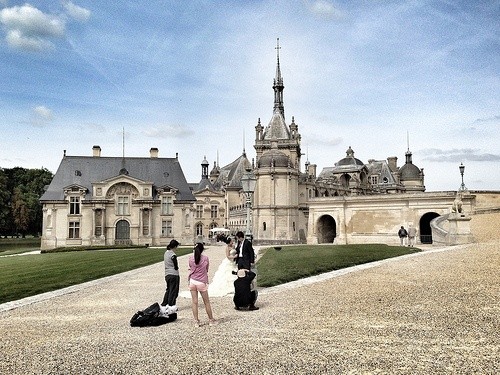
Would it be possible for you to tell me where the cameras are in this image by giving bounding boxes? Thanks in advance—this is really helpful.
[232,271,237,275]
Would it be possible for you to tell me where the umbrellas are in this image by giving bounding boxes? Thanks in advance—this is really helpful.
[208,227,230,233]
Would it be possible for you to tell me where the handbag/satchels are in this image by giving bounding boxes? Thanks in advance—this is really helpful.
[130,303,177,327]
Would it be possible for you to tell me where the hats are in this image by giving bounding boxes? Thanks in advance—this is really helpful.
[237,270,246,278]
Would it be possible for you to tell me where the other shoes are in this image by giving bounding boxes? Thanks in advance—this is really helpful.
[249,306,259,310]
[193,320,204,327]
[209,320,219,327]
[235,306,240,310]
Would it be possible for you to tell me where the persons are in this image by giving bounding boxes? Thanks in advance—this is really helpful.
[187,242,218,329]
[232,268,260,311]
[408,224,417,248]
[231,231,255,275]
[159,239,180,316]
[212,233,238,299]
[217,232,227,243]
[397,225,408,248]
